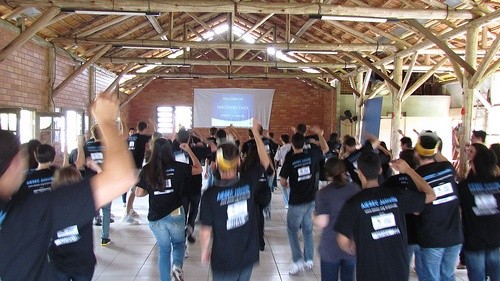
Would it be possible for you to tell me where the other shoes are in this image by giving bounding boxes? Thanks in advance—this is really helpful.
[95,219,102,226]
[185,245,189,258]
[110,219,114,223]
[289,260,304,275]
[457,264,467,269]
[304,260,313,269]
[172,266,185,281]
[125,218,138,225]
[185,230,195,242]
[131,211,140,217]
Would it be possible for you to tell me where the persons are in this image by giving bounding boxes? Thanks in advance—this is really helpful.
[0,92,500,281]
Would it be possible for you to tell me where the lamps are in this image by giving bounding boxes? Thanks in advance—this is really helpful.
[158,77,200,80]
[122,45,180,51]
[61,7,161,18]
[308,14,400,24]
[144,64,191,68]
[271,67,314,70]
[283,50,339,55]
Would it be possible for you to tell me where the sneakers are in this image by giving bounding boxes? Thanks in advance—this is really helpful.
[102,238,112,245]
